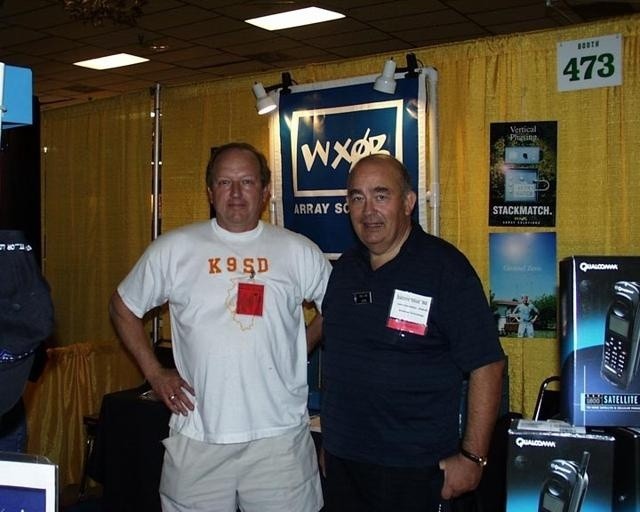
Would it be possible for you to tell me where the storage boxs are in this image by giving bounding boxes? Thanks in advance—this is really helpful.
[558,254,640,428]
[502,419,616,511]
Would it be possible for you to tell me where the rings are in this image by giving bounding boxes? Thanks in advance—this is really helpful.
[168,394,176,401]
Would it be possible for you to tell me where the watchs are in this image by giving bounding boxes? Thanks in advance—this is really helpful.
[460,447,488,467]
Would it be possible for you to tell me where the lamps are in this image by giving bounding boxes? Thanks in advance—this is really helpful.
[372,53,419,95]
[251,71,292,116]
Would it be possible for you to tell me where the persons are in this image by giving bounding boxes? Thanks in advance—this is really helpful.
[513,295,539,338]
[320,153,506,512]
[109,143,333,512]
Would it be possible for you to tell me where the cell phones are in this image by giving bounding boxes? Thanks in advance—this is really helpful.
[538,448,590,512]
[600,281,640,390]
